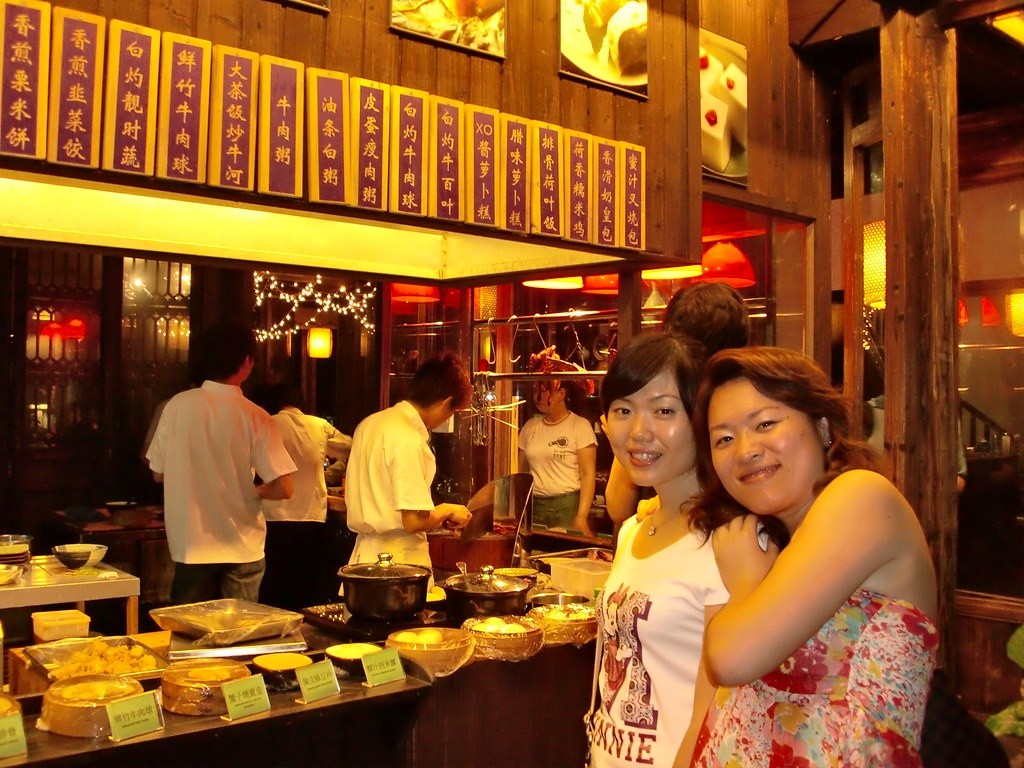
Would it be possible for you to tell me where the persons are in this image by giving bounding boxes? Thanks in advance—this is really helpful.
[606,282,967,530]
[49,400,99,444]
[673,346,946,768]
[518,371,598,536]
[337,350,474,602]
[584,333,769,768]
[249,383,354,610]
[145,322,298,605]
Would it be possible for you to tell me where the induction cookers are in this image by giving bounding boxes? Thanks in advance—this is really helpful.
[299,601,449,642]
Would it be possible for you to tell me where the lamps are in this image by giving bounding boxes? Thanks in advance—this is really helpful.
[523,241,757,311]
[307,318,333,359]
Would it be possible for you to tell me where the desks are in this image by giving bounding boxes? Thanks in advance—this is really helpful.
[966,447,1024,527]
[0,554,141,636]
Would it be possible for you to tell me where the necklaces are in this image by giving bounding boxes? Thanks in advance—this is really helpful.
[648,502,690,536]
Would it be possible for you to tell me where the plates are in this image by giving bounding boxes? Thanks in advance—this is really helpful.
[560,0,648,87]
[698,43,748,179]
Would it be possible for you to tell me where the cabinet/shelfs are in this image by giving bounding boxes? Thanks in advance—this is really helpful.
[59,509,179,607]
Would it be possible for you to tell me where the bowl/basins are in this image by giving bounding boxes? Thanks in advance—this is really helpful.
[105,501,138,516]
[0,533,34,566]
[252,653,314,690]
[530,592,591,608]
[0,564,23,584]
[52,543,108,571]
[325,644,384,677]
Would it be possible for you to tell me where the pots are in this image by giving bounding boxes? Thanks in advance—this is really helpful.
[442,564,529,627]
[337,553,432,623]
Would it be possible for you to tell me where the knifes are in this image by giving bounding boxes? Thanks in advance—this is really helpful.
[442,503,494,545]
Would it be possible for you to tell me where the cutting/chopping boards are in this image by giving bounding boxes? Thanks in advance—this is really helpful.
[425,527,516,572]
